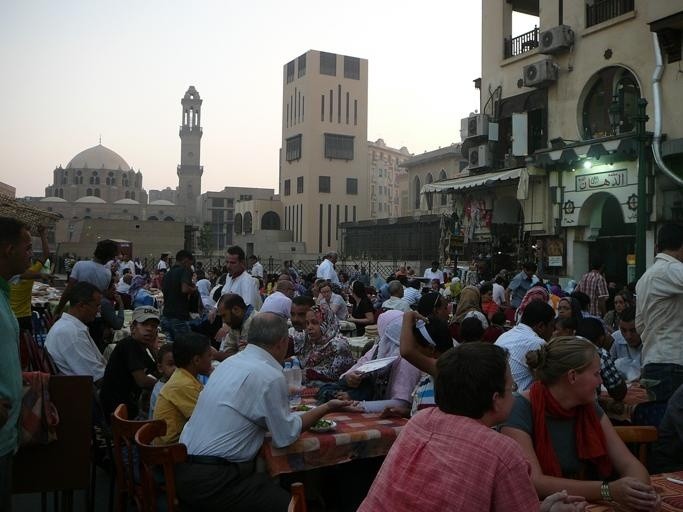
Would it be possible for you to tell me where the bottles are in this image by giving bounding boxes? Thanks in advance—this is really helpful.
[293,359,302,404]
[283,361,293,404]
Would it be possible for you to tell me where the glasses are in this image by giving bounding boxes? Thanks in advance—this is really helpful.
[505,381,519,395]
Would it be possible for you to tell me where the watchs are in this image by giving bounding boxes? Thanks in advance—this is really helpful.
[600,477,614,503]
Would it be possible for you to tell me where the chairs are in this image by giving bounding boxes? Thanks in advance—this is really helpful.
[24,329,38,371]
[115,294,124,309]
[22,374,125,512]
[32,311,44,347]
[42,314,51,332]
[612,426,658,468]
[113,402,166,512]
[286,482,307,512]
[134,422,188,512]
[44,309,54,326]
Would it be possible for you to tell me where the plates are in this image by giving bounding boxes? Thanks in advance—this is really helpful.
[289,406,316,415]
[309,419,337,429]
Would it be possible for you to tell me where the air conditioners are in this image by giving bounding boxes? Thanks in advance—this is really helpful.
[539,25,571,56]
[468,144,493,172]
[466,114,490,141]
[521,59,557,88]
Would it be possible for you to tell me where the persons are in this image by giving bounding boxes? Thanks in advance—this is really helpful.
[573,258,610,317]
[359,267,369,283]
[101,305,161,426]
[391,265,419,283]
[195,259,205,281]
[130,275,157,310]
[381,274,395,300]
[381,281,413,312]
[391,292,452,417]
[515,283,561,326]
[370,272,385,288]
[210,295,258,362]
[249,255,262,280]
[257,279,295,322]
[346,280,376,336]
[424,260,446,287]
[151,332,212,447]
[450,285,491,341]
[283,259,298,277]
[403,278,422,309]
[150,341,176,420]
[607,307,644,388]
[504,261,549,309]
[335,310,432,418]
[116,253,150,277]
[120,267,133,285]
[206,264,218,281]
[288,296,318,357]
[492,276,508,310]
[398,310,455,418]
[492,301,556,395]
[603,291,632,335]
[363,283,377,304]
[548,278,562,313]
[541,274,553,297]
[529,271,541,286]
[160,250,199,335]
[299,304,355,382]
[63,251,76,281]
[7,224,52,333]
[297,270,316,295]
[316,251,344,292]
[498,337,660,511]
[494,270,509,282]
[608,278,637,303]
[635,220,682,476]
[96,283,125,352]
[116,274,134,296]
[317,281,349,321]
[171,312,355,512]
[447,276,466,298]
[565,278,578,294]
[569,291,608,332]
[572,318,628,415]
[207,245,263,349]
[478,283,500,320]
[264,274,279,295]
[429,278,445,296]
[195,269,212,309]
[351,264,361,281]
[52,240,119,339]
[554,295,585,341]
[554,296,578,341]
[0,218,33,457]
[43,281,108,428]
[360,342,592,511]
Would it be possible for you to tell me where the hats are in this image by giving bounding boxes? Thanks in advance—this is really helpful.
[129,304,161,327]
